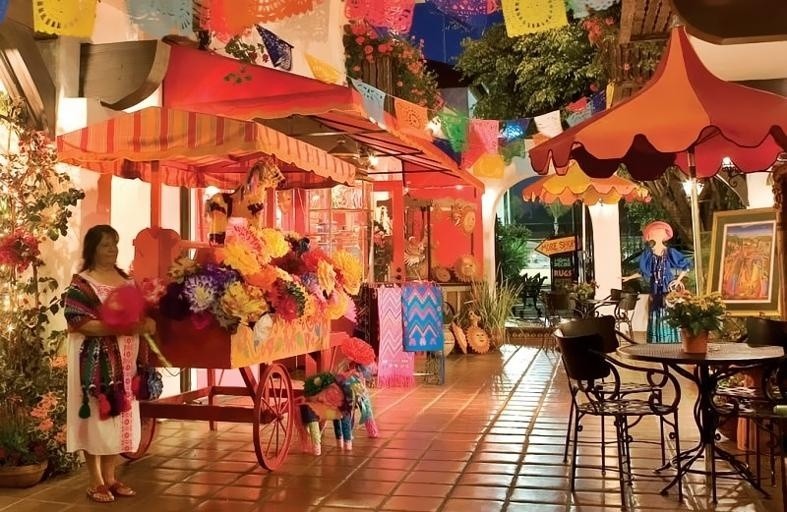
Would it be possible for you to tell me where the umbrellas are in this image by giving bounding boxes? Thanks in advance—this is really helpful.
[526,16,786,299]
[523,162,653,284]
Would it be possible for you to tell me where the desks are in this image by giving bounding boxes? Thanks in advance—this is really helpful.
[617,342,787,503]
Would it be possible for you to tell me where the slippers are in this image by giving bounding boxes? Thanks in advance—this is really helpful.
[85,481,137,502]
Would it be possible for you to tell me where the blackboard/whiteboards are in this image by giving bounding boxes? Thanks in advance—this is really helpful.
[550,251,578,292]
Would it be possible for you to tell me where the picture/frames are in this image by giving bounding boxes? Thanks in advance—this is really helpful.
[707,208,783,322]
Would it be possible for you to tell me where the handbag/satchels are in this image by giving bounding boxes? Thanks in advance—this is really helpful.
[138,363,163,402]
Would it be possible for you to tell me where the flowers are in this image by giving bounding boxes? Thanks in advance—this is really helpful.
[664,290,727,335]
[0,221,66,462]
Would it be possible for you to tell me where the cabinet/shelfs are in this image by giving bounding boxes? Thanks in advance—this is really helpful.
[355,280,483,384]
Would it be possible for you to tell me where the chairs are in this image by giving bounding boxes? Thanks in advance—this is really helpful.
[543,289,682,512]
[714,315,787,512]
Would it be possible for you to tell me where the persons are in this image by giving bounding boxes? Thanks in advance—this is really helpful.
[65,224,156,503]
[622,220,693,343]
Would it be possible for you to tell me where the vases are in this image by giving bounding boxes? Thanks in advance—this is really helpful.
[681,328,708,353]
[0,457,48,488]
